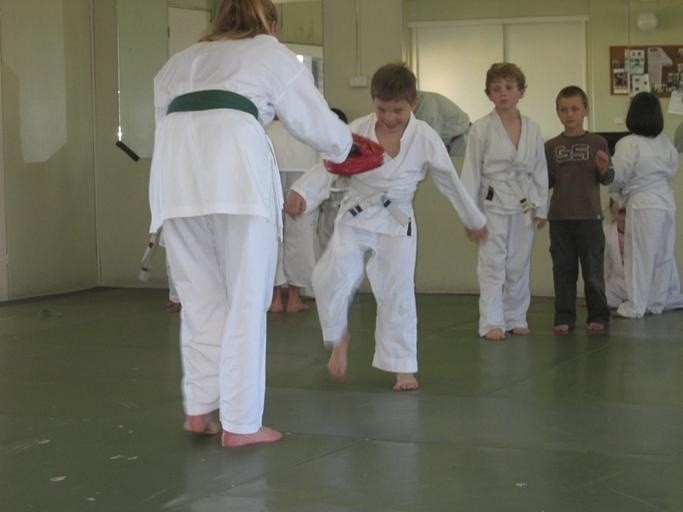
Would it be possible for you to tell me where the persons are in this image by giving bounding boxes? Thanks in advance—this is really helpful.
[316,106,351,258]
[460,62,550,340]
[156,229,181,314]
[262,110,316,313]
[610,91,679,319]
[282,59,489,392]
[409,88,474,159]
[147,2,387,448]
[544,85,615,333]
[578,196,682,310]
